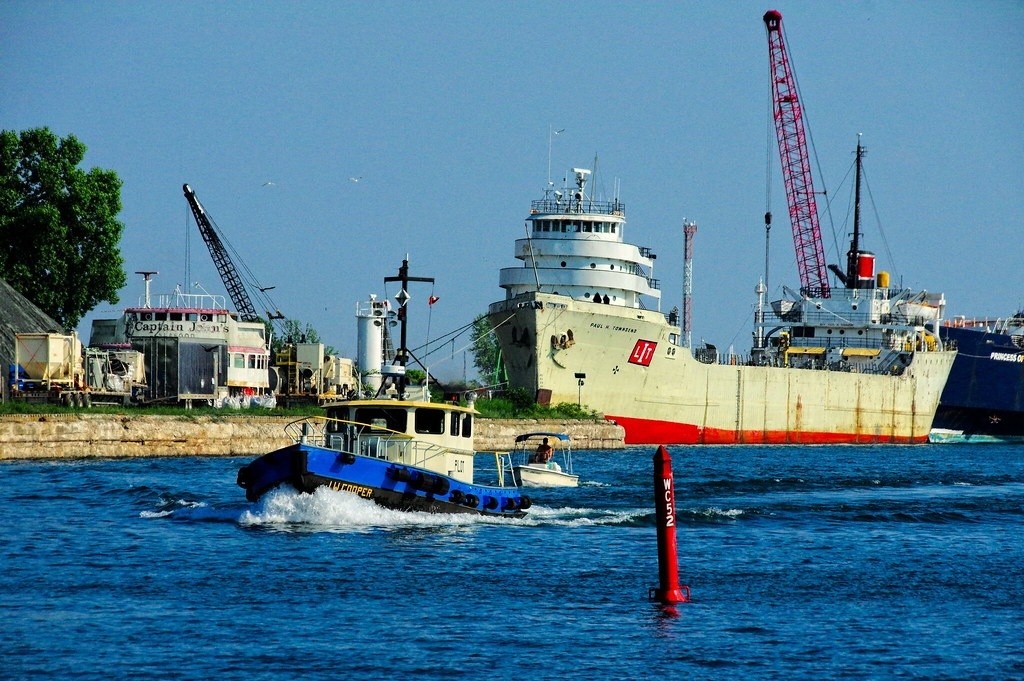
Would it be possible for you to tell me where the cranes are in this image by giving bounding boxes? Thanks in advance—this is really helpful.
[181,184,308,349]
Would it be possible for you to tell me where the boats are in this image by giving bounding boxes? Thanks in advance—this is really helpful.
[487,9,959,444]
[723,133,1024,437]
[503,432,579,488]
[237,259,532,518]
